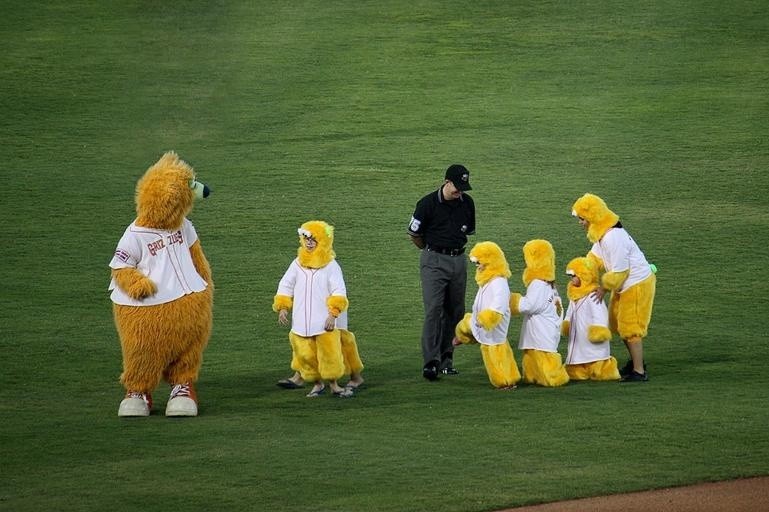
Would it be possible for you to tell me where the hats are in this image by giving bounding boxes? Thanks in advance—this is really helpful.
[445,164,473,190]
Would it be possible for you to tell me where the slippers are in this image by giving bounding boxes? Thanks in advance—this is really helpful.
[344,383,368,390]
[332,388,354,398]
[307,386,326,398]
[276,378,305,388]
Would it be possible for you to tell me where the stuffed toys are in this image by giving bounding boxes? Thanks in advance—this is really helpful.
[108,151,213,416]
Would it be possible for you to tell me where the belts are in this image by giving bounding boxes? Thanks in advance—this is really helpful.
[426,247,466,258]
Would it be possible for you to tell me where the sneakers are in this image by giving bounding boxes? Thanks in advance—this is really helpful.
[619,359,647,376]
[166,376,198,417]
[118,390,152,417]
[619,370,649,385]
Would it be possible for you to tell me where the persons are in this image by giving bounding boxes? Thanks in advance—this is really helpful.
[453,242,520,390]
[561,257,622,380]
[509,239,569,386]
[407,163,476,380]
[272,221,364,399]
[571,193,657,383]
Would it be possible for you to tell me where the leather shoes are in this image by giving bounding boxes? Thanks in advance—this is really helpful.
[422,365,438,381]
[441,368,459,374]
